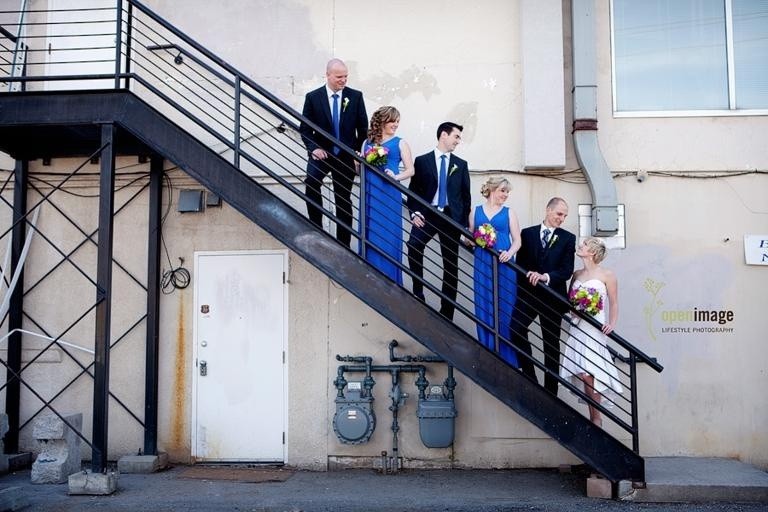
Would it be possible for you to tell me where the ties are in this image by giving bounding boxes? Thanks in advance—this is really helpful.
[438,154,447,213]
[332,93,342,156]
[542,229,551,250]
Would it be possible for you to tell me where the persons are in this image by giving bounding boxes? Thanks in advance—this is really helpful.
[407,122,471,321]
[557,237,617,428]
[355,107,415,288]
[300,59,368,248]
[510,198,576,397]
[466,176,522,368]
